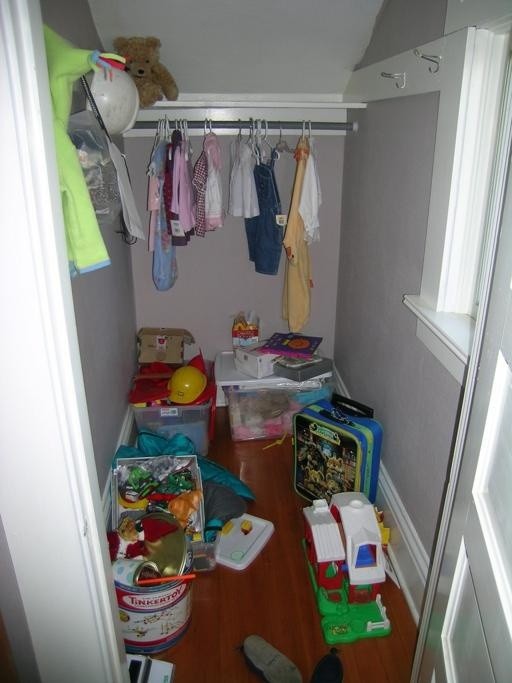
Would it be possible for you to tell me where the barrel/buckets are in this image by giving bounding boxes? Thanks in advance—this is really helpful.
[112,513,195,656]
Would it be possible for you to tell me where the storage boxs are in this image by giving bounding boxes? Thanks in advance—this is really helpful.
[223,372,336,440]
[129,359,209,460]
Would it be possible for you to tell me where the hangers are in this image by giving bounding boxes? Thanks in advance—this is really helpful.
[153,114,310,164]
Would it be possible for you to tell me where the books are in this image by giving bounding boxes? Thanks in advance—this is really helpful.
[262,333,323,358]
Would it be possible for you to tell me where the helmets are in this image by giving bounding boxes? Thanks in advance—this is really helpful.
[87,58,142,139]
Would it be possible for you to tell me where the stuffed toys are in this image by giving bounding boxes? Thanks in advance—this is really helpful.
[112,36,179,108]
